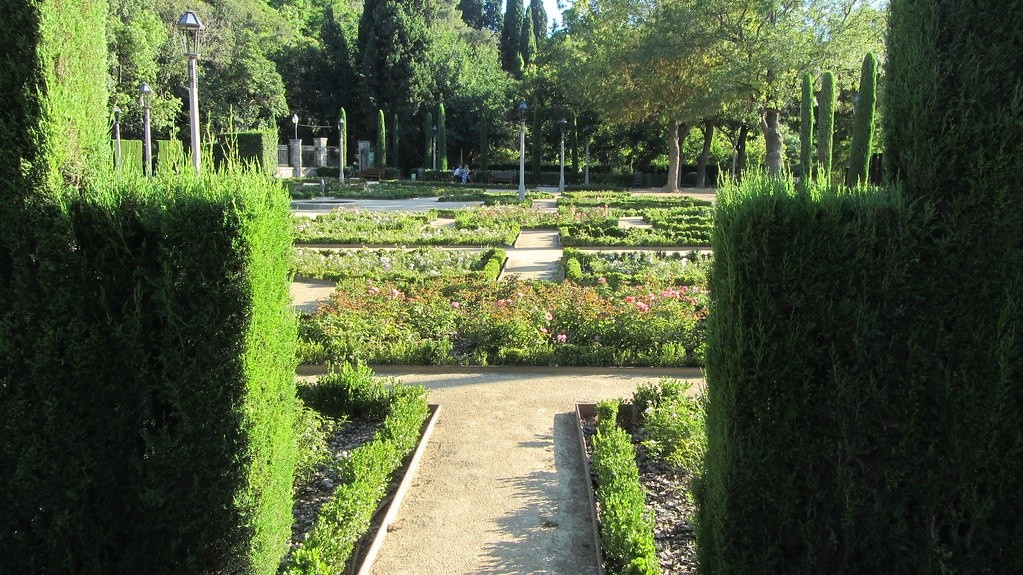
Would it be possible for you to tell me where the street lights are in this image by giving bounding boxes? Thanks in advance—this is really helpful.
[584,137,590,185]
[559,117,567,192]
[112,106,121,168]
[338,118,344,183]
[518,101,528,199]
[431,126,437,170]
[179,11,205,176]
[140,83,154,177]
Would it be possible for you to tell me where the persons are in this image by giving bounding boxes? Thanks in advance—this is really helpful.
[462,165,470,184]
[453,165,462,183]
